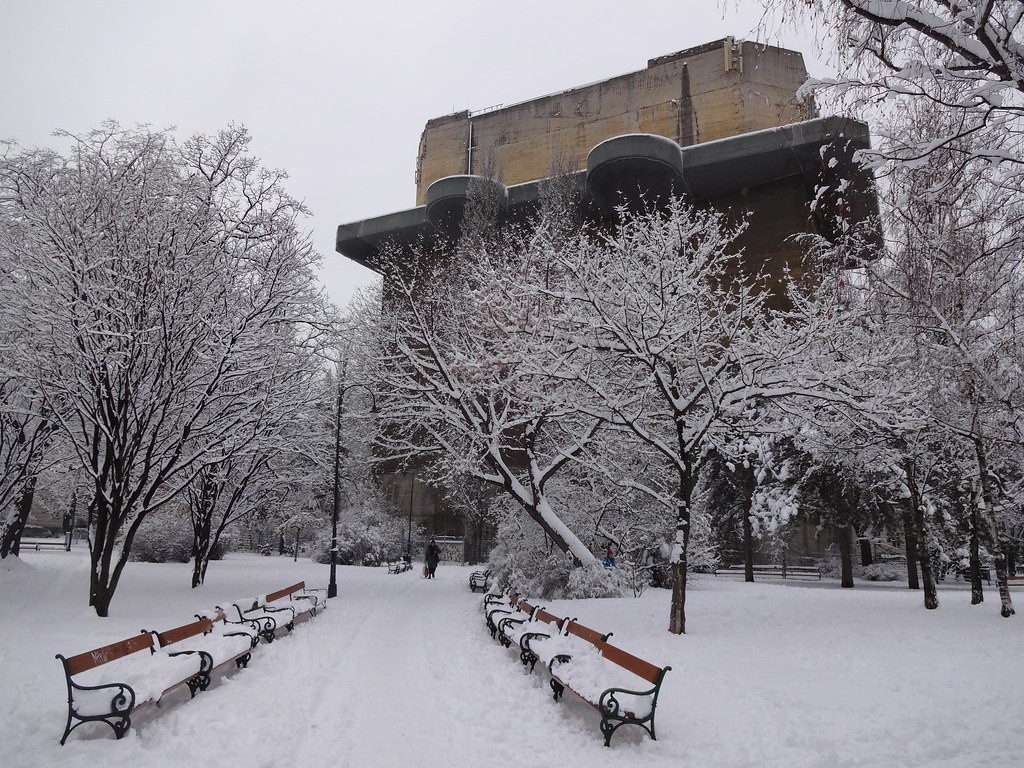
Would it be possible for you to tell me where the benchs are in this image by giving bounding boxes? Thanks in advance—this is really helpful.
[194,605,276,649]
[469,570,493,593]
[385,557,413,574]
[525,618,614,697]
[500,607,570,666]
[151,616,253,691]
[284,581,328,617]
[55,629,206,746]
[489,599,539,645]
[552,638,672,747]
[263,589,313,622]
[484,583,512,610]
[233,598,294,643]
[485,588,521,627]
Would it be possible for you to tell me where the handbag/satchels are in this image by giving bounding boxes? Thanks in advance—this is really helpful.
[422,564,425,576]
[424,564,428,578]
[435,554,439,563]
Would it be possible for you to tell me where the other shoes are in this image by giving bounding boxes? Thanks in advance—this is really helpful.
[432,573,435,578]
[427,577,431,579]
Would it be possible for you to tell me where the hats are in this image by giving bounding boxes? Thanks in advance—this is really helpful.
[430,539,435,544]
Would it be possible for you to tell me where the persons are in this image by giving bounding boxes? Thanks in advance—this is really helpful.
[606,546,615,565]
[425,540,442,579]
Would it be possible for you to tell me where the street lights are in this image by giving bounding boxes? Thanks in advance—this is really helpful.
[327,384,381,600]
